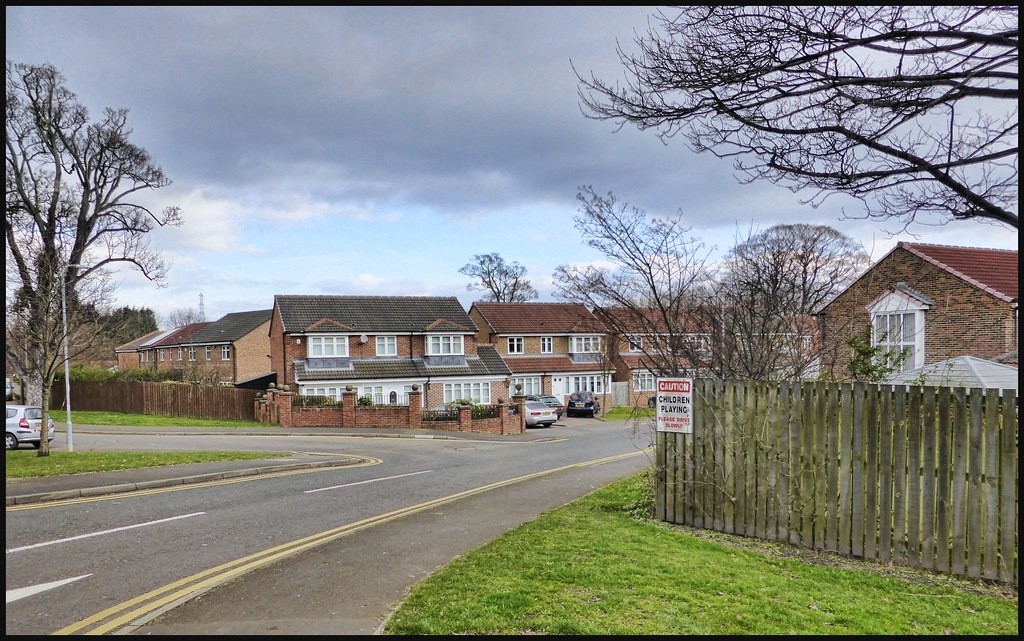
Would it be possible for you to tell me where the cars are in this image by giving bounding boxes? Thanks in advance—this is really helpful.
[6,377,14,401]
[509,401,558,429]
[566,391,601,419]
[647,395,656,408]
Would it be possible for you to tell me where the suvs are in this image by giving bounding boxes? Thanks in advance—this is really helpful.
[525,393,564,421]
[6,405,56,451]
